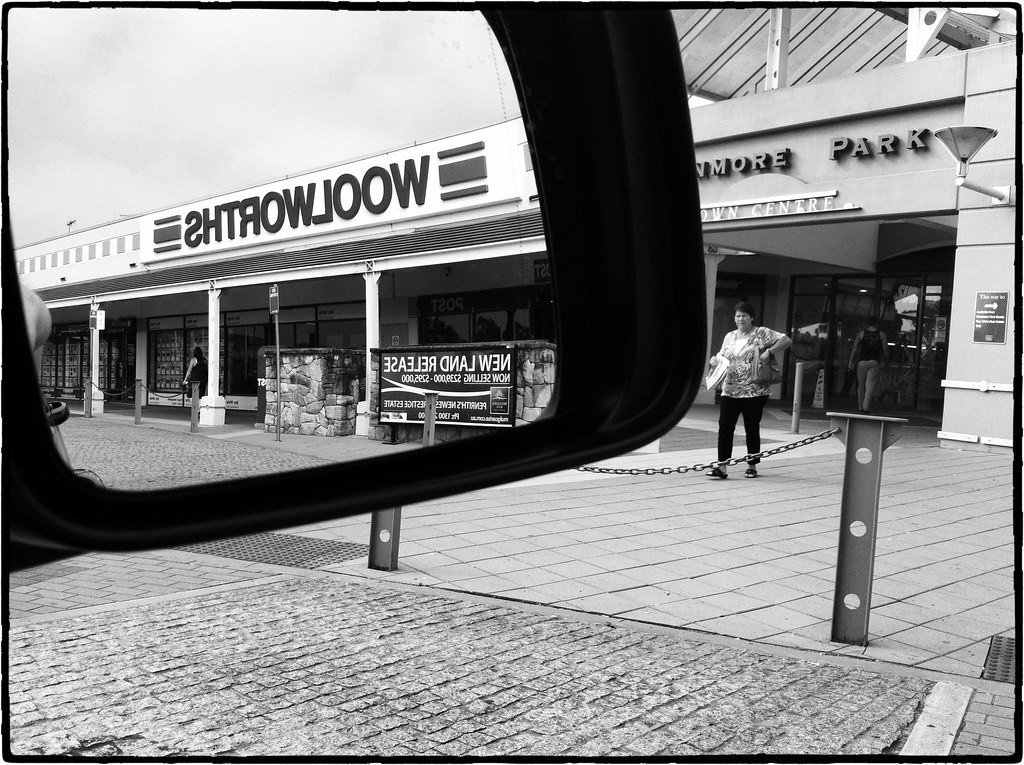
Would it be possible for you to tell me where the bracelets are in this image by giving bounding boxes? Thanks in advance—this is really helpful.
[766,348,772,357]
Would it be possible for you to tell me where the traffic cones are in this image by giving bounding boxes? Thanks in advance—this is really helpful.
[809,367,830,410]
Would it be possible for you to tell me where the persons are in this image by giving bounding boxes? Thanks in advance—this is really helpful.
[182,347,207,418]
[848,317,879,415]
[704,301,792,479]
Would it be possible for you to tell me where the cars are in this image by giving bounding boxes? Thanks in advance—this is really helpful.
[19,281,105,486]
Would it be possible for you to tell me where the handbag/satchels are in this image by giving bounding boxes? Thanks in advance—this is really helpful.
[751,327,783,384]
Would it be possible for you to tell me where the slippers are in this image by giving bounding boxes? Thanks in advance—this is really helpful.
[744,469,758,477]
[706,468,728,478]
